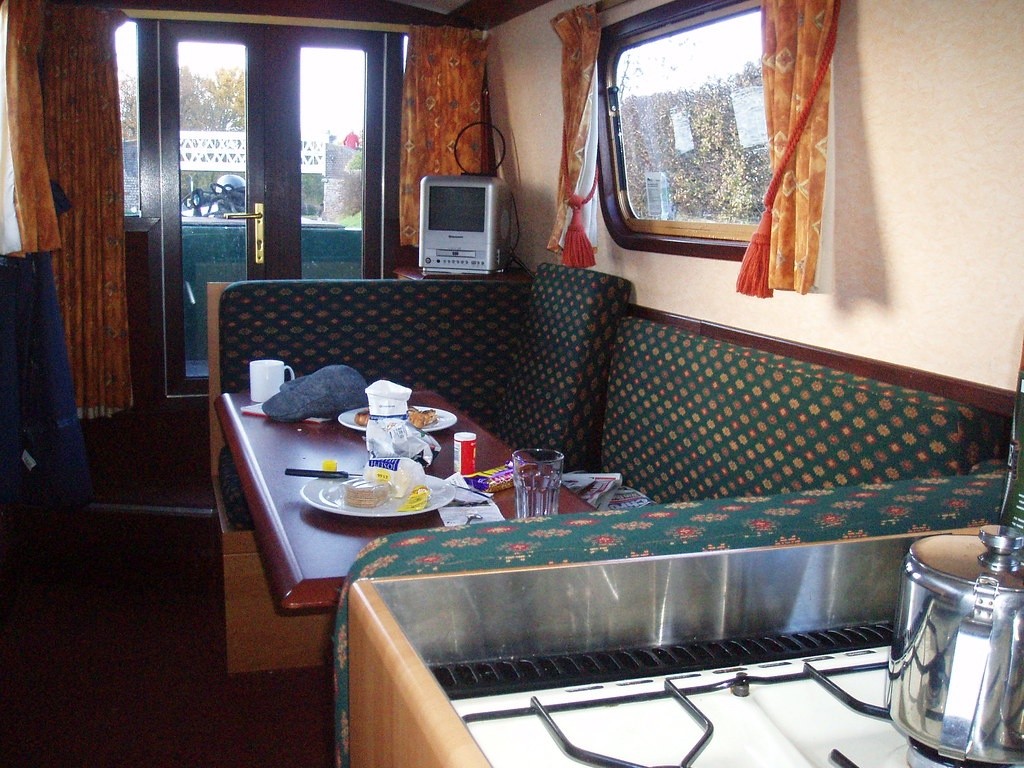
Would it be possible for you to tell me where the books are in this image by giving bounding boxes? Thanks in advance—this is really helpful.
[562,470,658,511]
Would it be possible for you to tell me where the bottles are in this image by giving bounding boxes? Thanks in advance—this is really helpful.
[454,431,477,475]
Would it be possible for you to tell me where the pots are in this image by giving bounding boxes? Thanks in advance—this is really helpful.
[882,521,1024,768]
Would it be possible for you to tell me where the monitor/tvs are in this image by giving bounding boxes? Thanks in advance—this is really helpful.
[419,176,510,273]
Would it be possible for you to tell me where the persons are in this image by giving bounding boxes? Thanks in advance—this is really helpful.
[343,129,360,150]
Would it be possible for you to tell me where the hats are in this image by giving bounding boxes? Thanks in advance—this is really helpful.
[262,365,369,423]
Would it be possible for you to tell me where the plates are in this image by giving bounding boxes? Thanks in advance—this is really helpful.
[338,406,458,433]
[300,472,457,518]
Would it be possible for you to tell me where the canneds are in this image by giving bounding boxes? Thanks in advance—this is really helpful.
[454,431,476,475]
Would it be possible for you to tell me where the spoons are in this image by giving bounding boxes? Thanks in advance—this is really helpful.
[464,514,483,526]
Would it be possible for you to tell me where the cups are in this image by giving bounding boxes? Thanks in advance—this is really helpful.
[249,359,295,403]
[512,448,564,519]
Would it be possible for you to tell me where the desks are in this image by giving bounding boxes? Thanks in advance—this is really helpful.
[215,390,596,611]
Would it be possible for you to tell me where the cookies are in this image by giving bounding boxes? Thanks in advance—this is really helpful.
[344,478,390,509]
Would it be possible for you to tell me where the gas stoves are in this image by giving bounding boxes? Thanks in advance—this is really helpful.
[347,527,1023,765]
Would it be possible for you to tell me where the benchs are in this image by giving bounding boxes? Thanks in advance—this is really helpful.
[205,264,1023,768]
